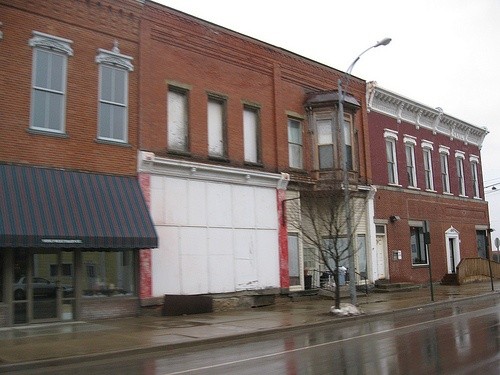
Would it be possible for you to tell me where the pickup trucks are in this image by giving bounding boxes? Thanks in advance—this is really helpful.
[13,275,63,300]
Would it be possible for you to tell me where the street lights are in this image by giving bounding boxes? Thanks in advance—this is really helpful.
[483,228,494,292]
[335,37,394,307]
